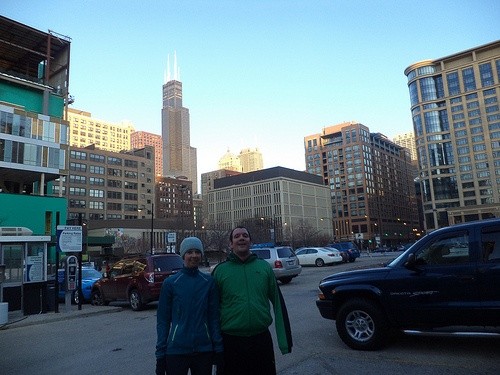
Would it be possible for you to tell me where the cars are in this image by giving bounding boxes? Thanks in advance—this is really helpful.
[294,247,342,267]
[58,267,101,305]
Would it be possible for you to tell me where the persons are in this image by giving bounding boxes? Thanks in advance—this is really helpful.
[211,226,293,375]
[98,262,110,272]
[155,237,224,375]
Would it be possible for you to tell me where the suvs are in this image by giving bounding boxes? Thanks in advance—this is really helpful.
[324,242,360,262]
[249,245,302,284]
[91,253,186,312]
[315,218,500,352]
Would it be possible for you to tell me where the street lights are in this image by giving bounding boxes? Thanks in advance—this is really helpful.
[320,217,335,242]
[202,226,206,240]
[138,203,154,254]
[261,214,275,242]
[281,219,287,244]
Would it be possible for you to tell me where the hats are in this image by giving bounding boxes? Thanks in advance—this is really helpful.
[180,236,204,260]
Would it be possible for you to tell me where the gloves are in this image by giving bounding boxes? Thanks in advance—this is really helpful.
[155,358,166,375]
[214,352,223,367]
